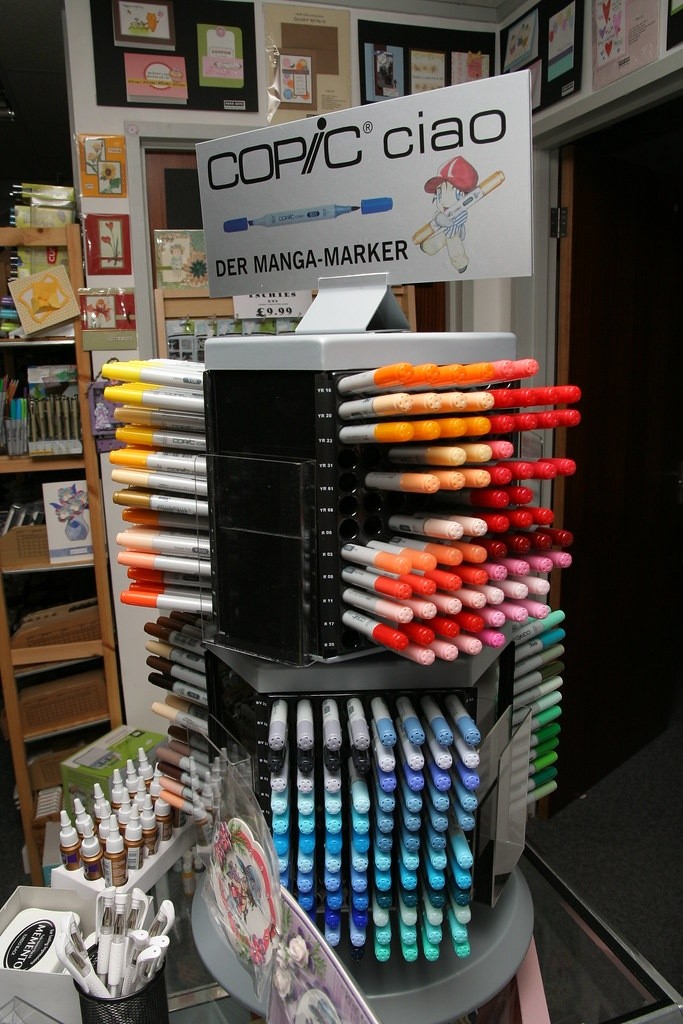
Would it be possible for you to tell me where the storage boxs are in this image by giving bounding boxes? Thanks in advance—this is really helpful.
[1,886,101,1021]
[16,180,76,276]
[154,230,204,289]
[61,725,169,819]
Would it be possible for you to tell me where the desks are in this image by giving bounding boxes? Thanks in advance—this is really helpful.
[193,865,554,1024]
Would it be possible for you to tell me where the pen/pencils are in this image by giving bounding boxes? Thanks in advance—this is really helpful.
[0,373,79,456]
[0,184,24,333]
[54,885,176,1024]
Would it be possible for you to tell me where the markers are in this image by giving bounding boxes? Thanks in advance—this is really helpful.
[270,357,581,961]
[102,357,210,825]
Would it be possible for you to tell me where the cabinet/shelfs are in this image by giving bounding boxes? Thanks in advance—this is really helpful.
[0,225,123,891]
